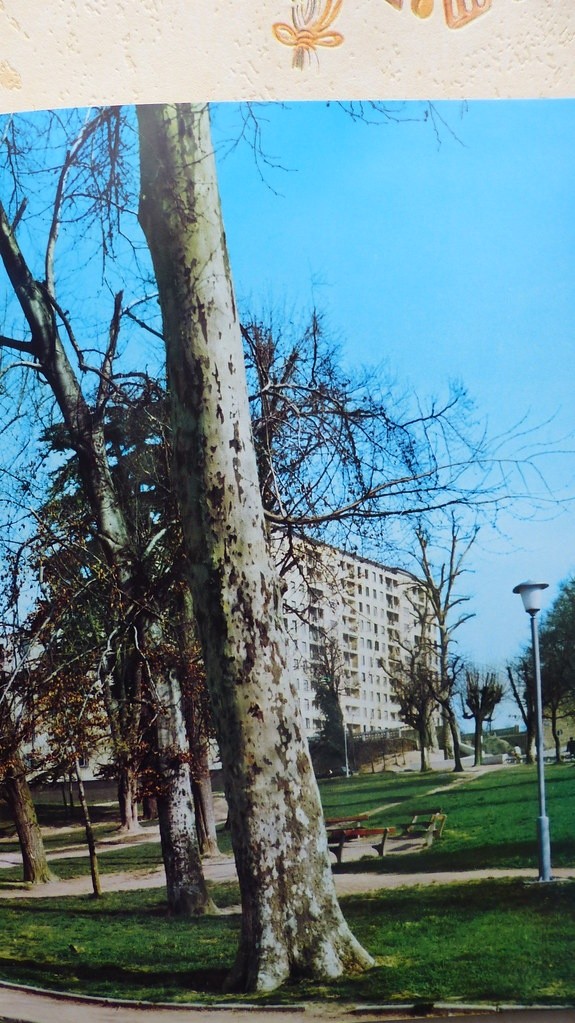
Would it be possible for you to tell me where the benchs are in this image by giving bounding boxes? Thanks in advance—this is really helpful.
[323,813,396,862]
[395,806,447,840]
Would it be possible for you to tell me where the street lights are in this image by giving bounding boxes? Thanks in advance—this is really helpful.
[510,577,553,882]
